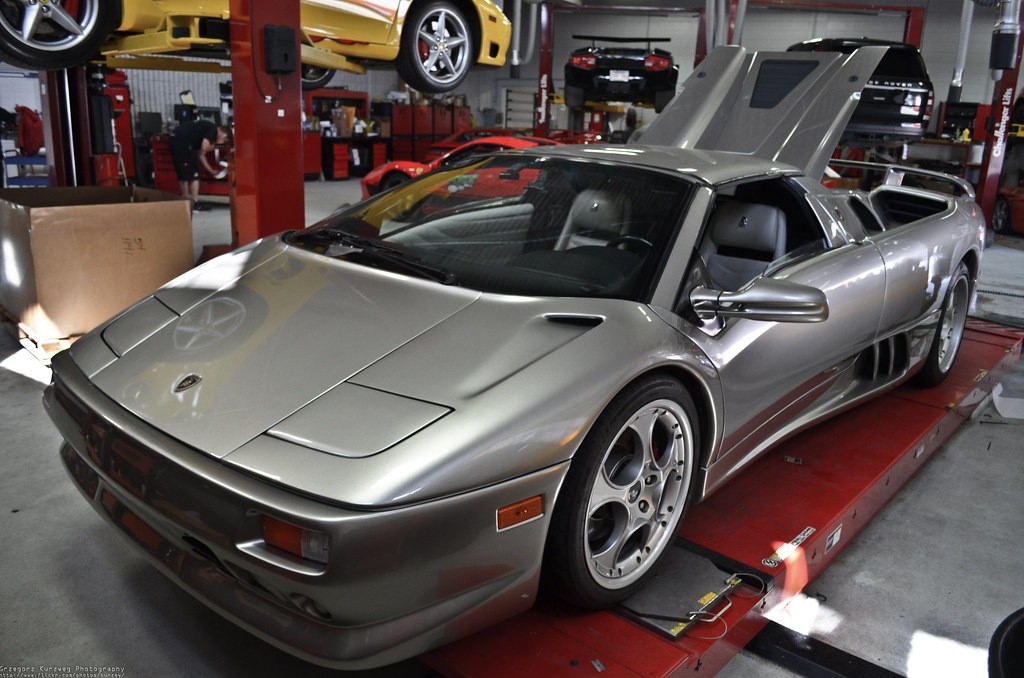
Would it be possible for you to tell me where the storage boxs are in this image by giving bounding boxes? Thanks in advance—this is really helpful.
[0,185,195,342]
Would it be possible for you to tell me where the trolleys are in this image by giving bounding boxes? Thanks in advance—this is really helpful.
[3,148,50,188]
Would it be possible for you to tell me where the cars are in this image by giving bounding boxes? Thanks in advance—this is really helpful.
[360,136,565,199]
[563,34,679,115]
[42,45,985,671]
[0,1,512,92]
[786,37,937,149]
[424,128,629,164]
[991,180,1023,233]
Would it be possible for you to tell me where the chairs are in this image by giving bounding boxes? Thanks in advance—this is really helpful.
[675,200,788,315]
[554,190,632,249]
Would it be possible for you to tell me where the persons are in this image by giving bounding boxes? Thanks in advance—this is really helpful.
[166,119,235,213]
